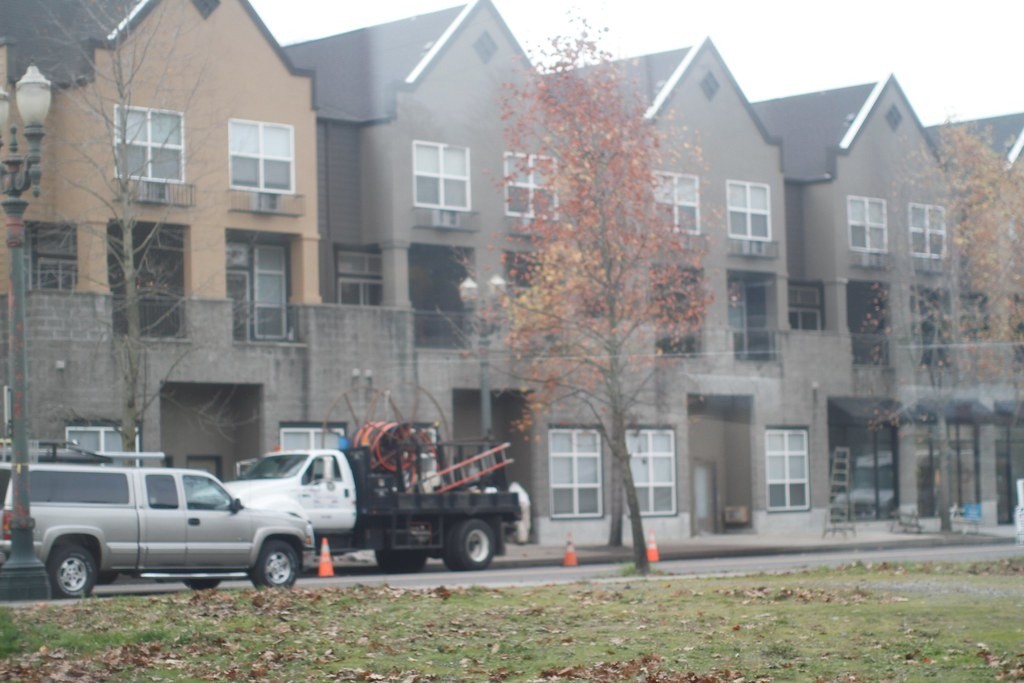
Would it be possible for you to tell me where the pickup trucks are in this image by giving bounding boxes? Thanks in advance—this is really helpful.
[189,444,523,574]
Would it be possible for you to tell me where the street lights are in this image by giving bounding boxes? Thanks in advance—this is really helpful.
[459,273,508,438]
[0,33,53,602]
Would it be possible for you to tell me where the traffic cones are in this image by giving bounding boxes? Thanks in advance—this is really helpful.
[560,531,580,566]
[645,528,659,564]
[314,536,337,579]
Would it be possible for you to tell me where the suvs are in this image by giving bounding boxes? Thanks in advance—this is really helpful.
[0,460,314,600]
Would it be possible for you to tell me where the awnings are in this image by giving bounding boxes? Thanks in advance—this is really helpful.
[829,397,917,427]
[995,401,1024,428]
[915,400,992,424]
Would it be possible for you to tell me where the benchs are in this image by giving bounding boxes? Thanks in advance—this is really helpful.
[889,510,925,535]
[950,518,982,536]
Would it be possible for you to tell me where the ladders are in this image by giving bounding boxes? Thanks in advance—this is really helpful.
[820,446,859,539]
[404,441,515,493]
